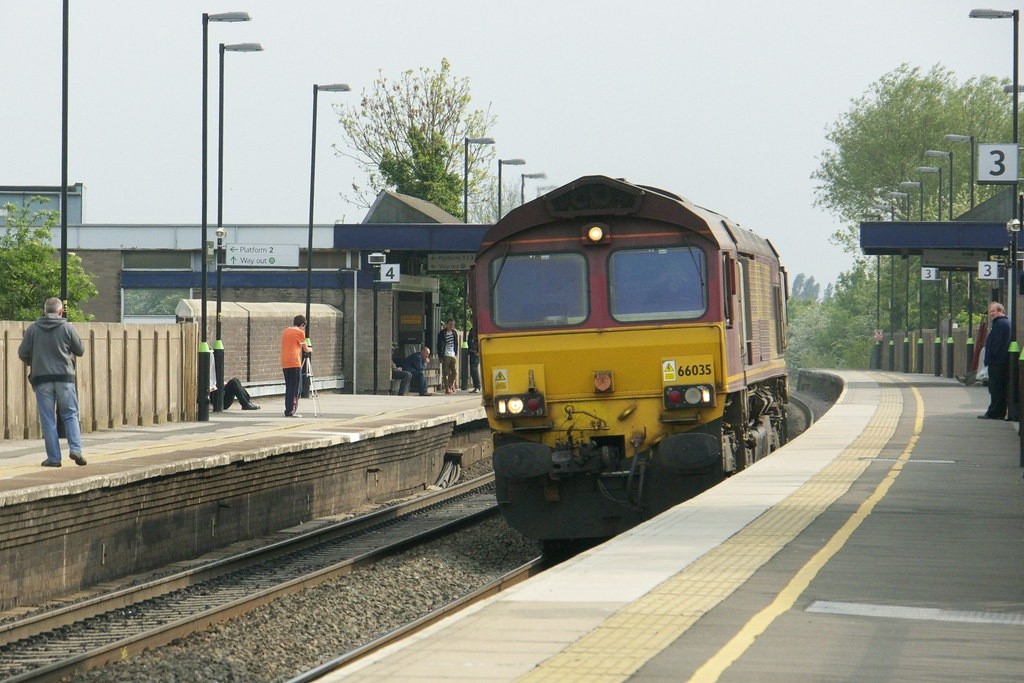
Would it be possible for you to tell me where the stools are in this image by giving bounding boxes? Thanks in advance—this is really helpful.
[390,378,401,396]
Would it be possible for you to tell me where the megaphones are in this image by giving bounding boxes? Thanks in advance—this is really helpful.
[1007,219,1021,232]
[215,228,225,238]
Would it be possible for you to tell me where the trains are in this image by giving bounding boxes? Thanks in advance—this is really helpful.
[469,173,791,544]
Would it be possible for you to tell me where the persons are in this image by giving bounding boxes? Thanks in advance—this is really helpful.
[400,347,432,396]
[391,346,413,396]
[467,314,481,393]
[977,302,1012,419]
[437,318,458,394]
[211,378,260,409]
[279,314,312,418]
[17,296,89,467]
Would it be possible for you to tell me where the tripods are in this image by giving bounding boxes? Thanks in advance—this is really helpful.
[301,352,323,418]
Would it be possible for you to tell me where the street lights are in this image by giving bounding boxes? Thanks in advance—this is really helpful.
[943,133,974,376]
[502,158,528,223]
[216,41,266,412]
[899,179,925,374]
[967,8,1024,422]
[301,82,354,398]
[924,149,956,381]
[521,173,545,206]
[461,137,496,222]
[866,190,911,375]
[193,10,251,420]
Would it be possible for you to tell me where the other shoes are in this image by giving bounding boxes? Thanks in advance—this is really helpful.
[69,450,87,465]
[470,388,479,393]
[446,385,455,395]
[40,458,61,467]
[978,415,1006,420]
[290,413,301,418]
[420,391,433,397]
[242,401,260,410]
